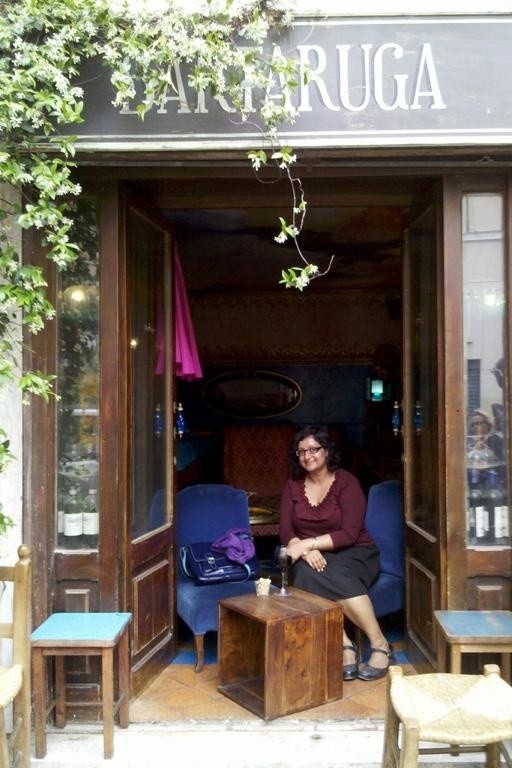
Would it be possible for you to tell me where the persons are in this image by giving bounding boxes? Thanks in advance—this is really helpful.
[364,346,401,458]
[470,411,507,489]
[278,424,396,682]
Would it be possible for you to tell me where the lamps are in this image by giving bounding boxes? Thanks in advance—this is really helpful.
[355,480,403,663]
[175,484,280,673]
[0,544,32,768]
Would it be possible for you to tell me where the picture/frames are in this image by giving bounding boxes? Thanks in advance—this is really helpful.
[216,586,344,720]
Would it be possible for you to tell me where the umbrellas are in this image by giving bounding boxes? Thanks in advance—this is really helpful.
[357,648,393,681]
[342,640,359,680]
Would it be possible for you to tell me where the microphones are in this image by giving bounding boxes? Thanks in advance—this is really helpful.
[382,665,511,767]
[433,609,512,756]
[33,612,133,760]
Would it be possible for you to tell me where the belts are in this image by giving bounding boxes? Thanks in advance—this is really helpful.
[273,544,293,597]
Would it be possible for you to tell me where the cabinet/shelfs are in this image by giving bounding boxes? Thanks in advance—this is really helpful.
[468,467,509,545]
[55,487,99,552]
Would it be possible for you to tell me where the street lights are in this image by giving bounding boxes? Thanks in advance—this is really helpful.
[179,542,257,586]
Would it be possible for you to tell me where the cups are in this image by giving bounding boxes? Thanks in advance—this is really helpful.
[469,421,486,427]
[295,446,324,457]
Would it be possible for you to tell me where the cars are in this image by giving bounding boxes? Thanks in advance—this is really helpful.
[254,579,272,600]
